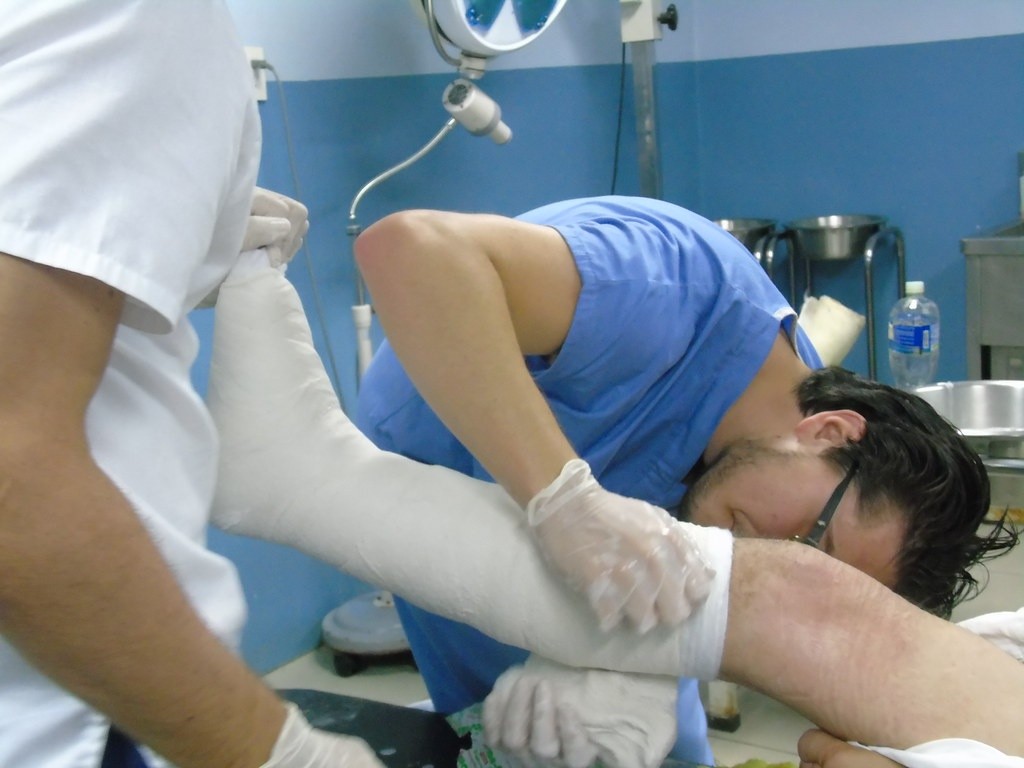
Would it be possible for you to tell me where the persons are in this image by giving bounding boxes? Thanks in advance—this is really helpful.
[355,194,990,768]
[1,0,386,768]
[207,247,1022,768]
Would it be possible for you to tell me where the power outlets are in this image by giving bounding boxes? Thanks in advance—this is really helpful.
[243,45,266,100]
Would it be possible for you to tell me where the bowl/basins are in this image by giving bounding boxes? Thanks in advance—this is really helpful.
[712,219,776,245]
[782,214,888,262]
[906,381,1024,454]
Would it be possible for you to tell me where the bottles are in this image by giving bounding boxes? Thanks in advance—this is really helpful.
[887,281,939,392]
